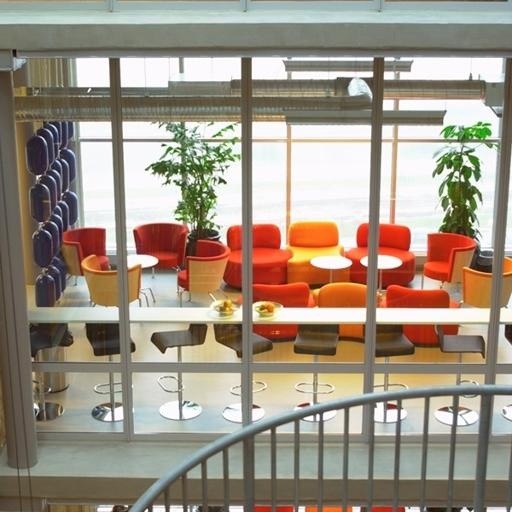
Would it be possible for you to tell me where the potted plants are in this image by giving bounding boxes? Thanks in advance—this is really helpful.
[150,121,243,259]
[432,122,494,275]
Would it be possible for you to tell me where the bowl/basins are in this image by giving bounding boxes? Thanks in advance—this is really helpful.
[211,300,241,316]
[253,300,283,317]
